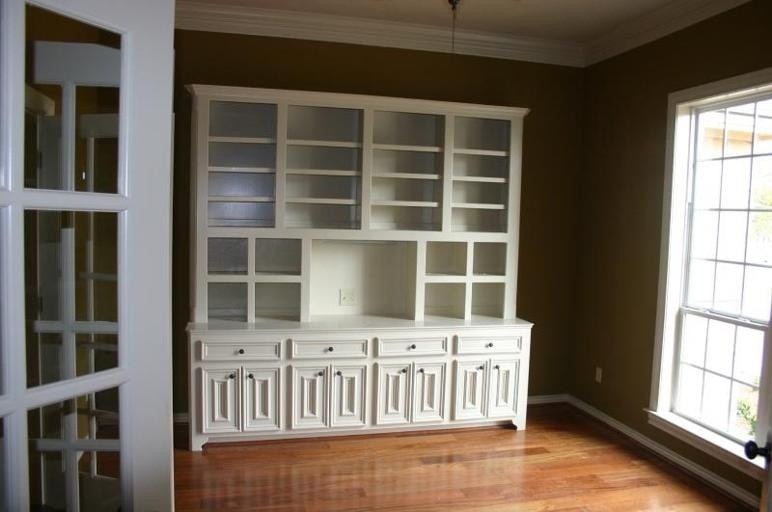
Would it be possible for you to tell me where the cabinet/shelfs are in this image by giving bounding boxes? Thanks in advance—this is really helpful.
[182,80,538,449]
